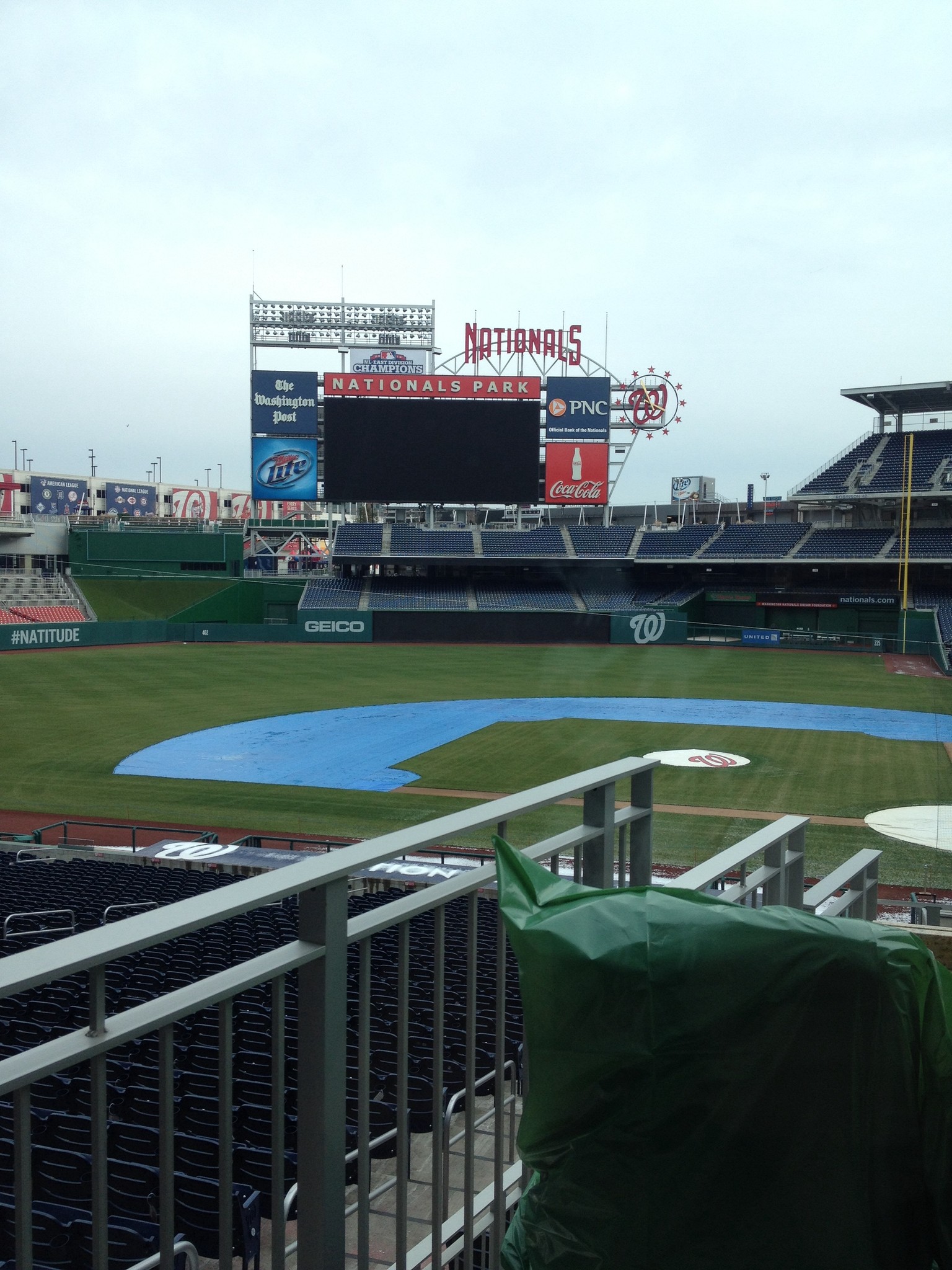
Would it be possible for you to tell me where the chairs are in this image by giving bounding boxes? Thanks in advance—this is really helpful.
[0,849,522,1270]
[300,429,952,669]
